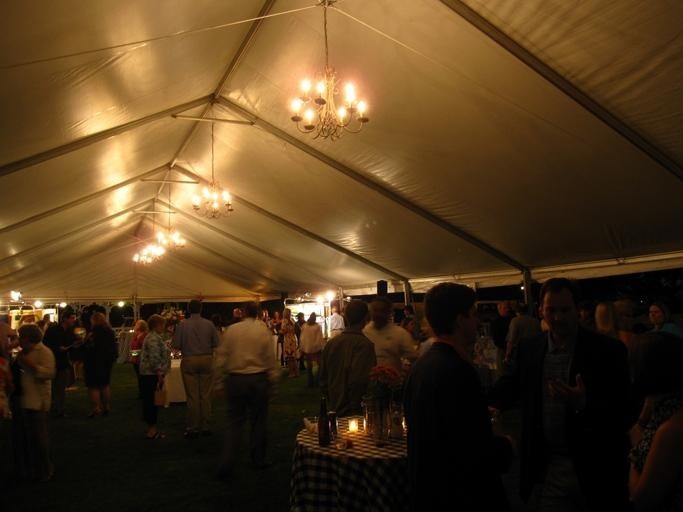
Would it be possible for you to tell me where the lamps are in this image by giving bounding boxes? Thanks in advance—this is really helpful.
[288,0,369,141]
[193,106,233,219]
[133,171,185,265]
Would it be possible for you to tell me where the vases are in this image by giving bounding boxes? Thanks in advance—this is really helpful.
[371,400,389,447]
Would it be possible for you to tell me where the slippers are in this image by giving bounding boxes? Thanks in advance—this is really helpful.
[144,430,166,440]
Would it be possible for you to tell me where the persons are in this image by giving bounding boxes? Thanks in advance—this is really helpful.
[0,279,683,512]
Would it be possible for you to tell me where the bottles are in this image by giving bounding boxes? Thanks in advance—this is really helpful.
[318,397,330,447]
[328,412,337,433]
[391,403,402,438]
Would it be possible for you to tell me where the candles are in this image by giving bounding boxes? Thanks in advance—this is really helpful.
[348,419,358,432]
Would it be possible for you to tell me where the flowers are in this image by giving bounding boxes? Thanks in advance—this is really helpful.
[367,360,407,435]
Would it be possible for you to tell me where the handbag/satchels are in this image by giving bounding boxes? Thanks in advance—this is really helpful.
[153,381,168,407]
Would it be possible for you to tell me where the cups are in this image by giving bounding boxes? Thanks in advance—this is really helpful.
[307,420,318,437]
[303,417,318,435]
[348,418,359,432]
